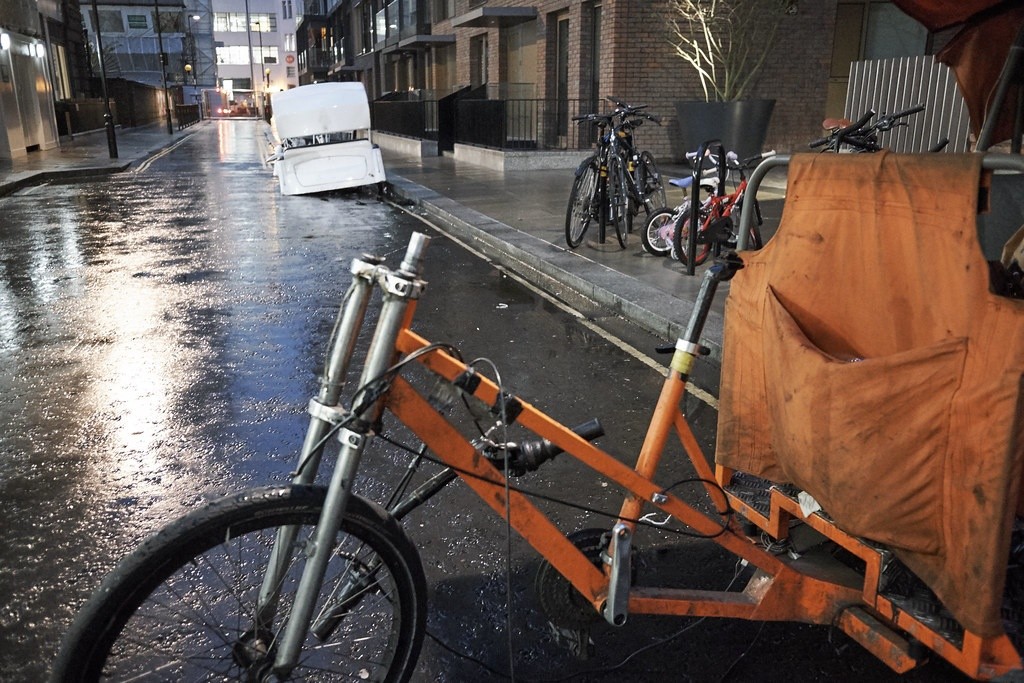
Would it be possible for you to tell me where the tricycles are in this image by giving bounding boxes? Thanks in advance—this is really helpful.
[46,148,1024,683]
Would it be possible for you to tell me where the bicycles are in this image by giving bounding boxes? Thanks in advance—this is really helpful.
[809,101,950,155]
[563,96,777,267]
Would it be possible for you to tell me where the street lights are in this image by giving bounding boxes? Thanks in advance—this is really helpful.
[255,22,265,93]
[184,14,202,118]
[265,68,270,120]
